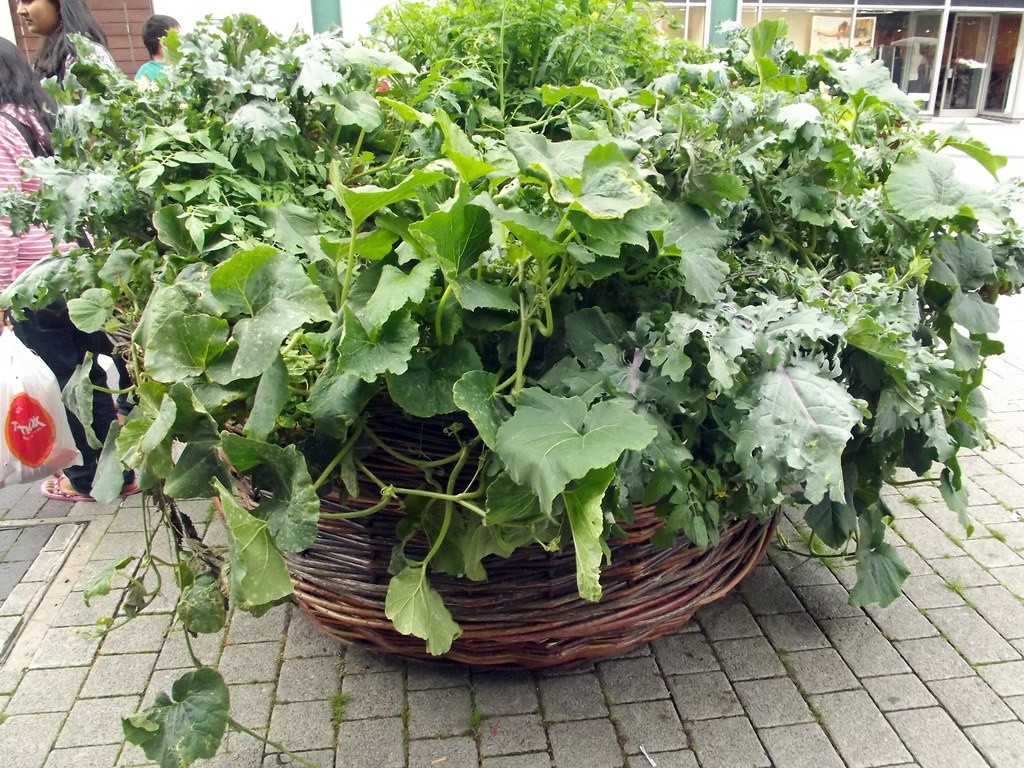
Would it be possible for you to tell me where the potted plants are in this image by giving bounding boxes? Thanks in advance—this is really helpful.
[2,0,1023,766]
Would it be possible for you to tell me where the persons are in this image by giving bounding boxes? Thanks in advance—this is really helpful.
[0,1,184,505]
[813,21,850,51]
[892,44,931,92]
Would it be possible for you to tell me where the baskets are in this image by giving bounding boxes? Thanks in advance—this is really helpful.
[213,398,783,665]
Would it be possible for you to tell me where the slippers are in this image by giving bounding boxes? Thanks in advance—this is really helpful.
[41,469,142,502]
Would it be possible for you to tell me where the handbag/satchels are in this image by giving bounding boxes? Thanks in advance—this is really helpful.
[0,326,84,490]
